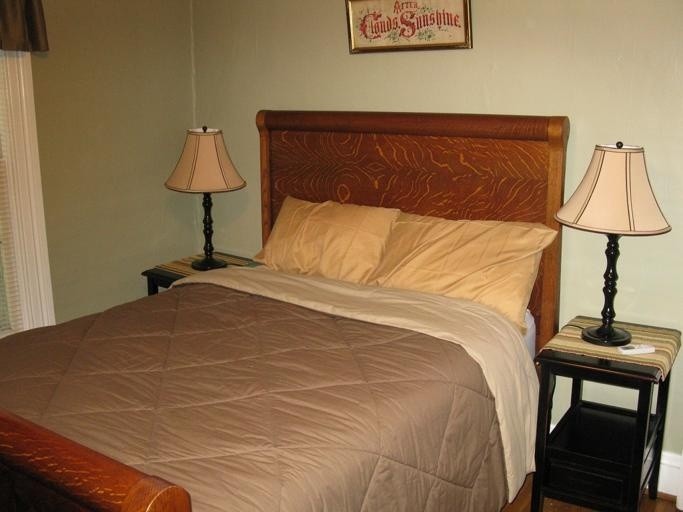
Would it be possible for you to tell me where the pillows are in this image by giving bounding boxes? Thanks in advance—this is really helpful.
[252,195,400,286]
[363,213,558,335]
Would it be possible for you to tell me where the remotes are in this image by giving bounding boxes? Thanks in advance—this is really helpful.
[617,343,656,355]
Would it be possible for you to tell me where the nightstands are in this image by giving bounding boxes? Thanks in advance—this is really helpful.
[138,251,260,297]
[529,315,681,512]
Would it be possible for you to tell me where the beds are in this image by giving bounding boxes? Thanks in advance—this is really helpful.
[1,108,571,512]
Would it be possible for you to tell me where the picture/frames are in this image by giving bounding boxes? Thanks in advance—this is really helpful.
[344,0,474,54]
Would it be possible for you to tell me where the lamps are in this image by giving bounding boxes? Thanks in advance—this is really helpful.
[164,122,246,272]
[554,140,672,347]
